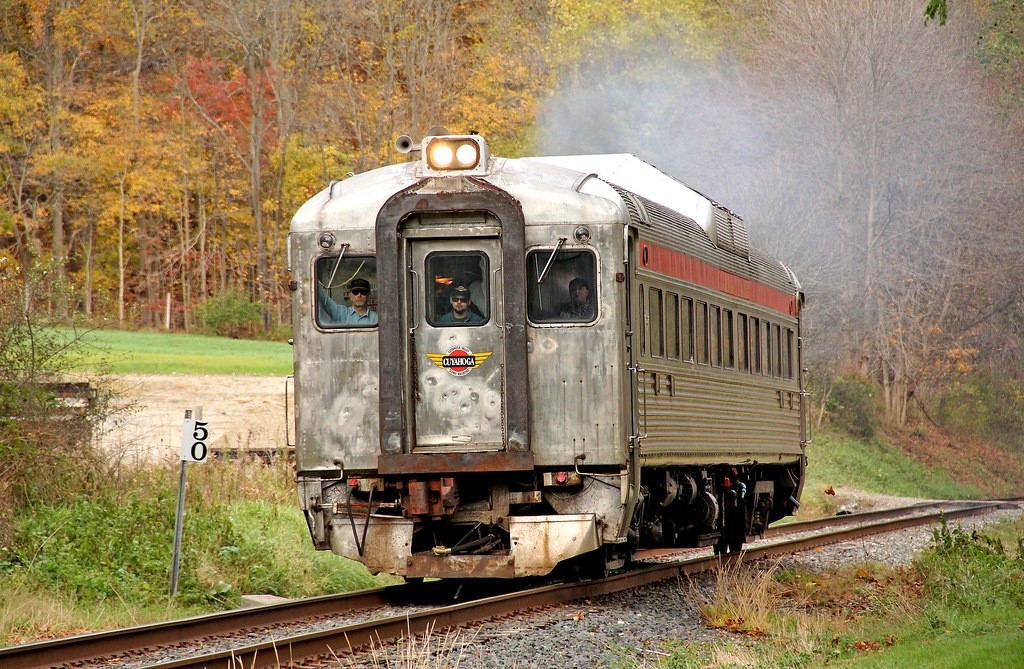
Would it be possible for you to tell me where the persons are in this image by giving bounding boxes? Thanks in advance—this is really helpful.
[437,284,482,321]
[317,276,379,326]
[555,276,593,321]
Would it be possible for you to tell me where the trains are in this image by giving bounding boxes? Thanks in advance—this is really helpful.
[285,126,812,584]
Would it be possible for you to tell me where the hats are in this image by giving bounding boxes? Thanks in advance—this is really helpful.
[350,278,370,289]
[451,286,470,298]
[569,277,586,292]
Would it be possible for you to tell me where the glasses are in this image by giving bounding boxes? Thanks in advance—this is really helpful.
[350,288,369,295]
[452,297,467,303]
